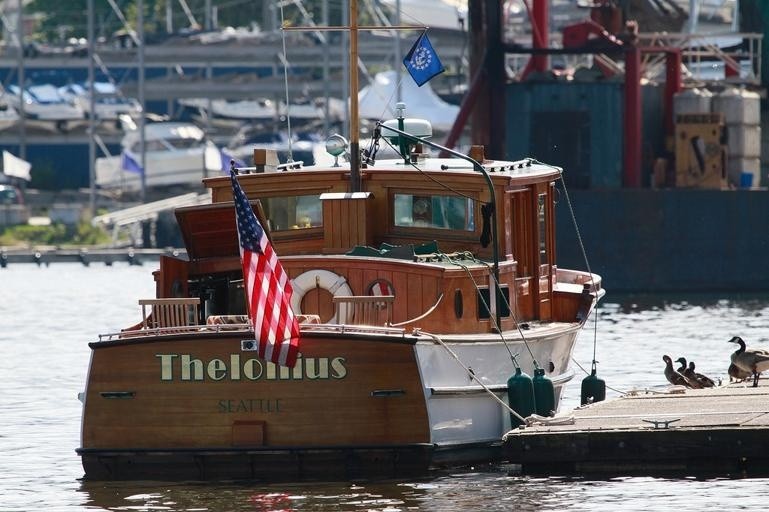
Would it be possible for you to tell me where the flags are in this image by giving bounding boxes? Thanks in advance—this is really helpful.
[402,29,444,87]
[230,168,301,370]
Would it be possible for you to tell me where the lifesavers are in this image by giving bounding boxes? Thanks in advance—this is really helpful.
[290,270,354,324]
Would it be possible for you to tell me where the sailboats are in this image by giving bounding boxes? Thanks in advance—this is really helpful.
[77,1,606,478]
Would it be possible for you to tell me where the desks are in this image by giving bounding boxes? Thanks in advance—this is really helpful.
[208,313,321,332]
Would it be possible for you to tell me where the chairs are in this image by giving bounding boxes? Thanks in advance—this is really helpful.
[331,295,395,326]
[138,298,202,337]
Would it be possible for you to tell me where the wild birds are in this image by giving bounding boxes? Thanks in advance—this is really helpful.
[663,355,691,387]
[674,357,715,387]
[728,336,769,387]
[685,362,704,389]
[728,348,769,383]
